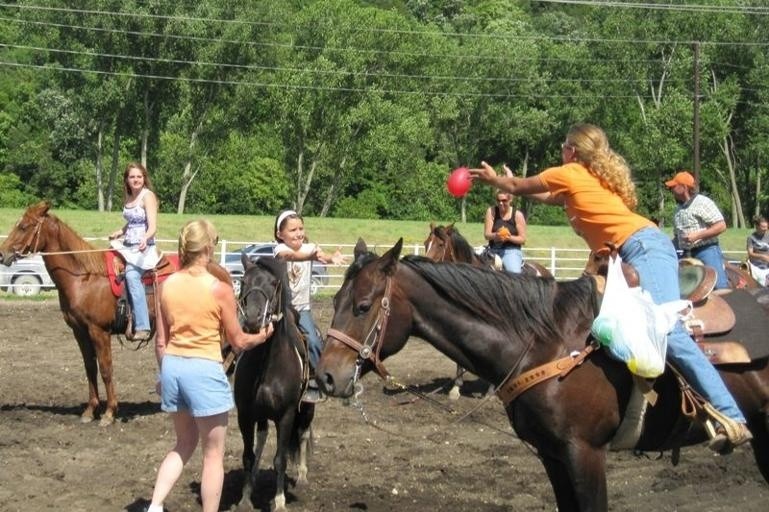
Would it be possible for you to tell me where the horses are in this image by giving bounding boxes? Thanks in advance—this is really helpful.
[0,199,238,428]
[233,249,325,512]
[313,237,769,512]
[581,248,763,292]
[422,220,556,401]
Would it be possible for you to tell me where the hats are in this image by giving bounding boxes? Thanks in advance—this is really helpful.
[665,171,697,189]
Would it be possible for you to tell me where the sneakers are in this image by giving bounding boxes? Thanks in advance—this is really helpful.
[133,330,150,340]
[712,424,751,452]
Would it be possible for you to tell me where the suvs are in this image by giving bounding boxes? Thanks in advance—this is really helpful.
[214,238,332,298]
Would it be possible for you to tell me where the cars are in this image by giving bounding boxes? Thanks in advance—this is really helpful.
[0,248,55,296]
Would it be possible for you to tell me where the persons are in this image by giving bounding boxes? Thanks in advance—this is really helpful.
[148,220,277,512]
[746,214,769,288]
[467,122,753,451]
[661,171,728,290]
[272,210,351,388]
[109,163,157,339]
[485,190,524,275]
